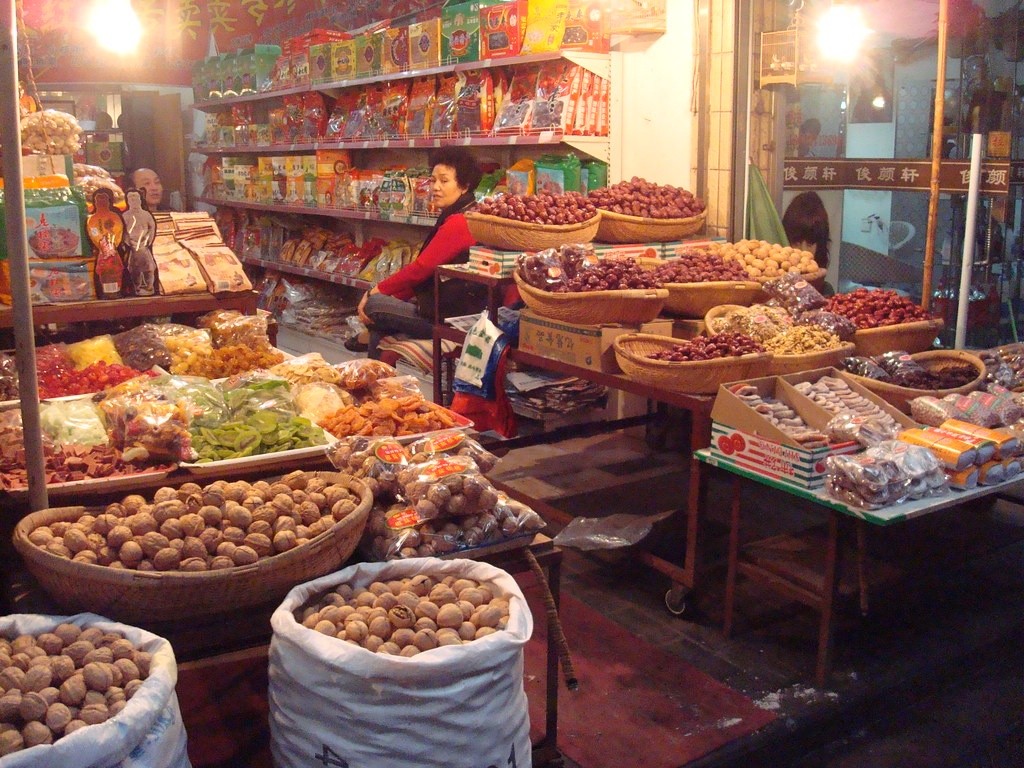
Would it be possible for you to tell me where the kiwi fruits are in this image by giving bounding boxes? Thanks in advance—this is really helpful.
[176,374,328,463]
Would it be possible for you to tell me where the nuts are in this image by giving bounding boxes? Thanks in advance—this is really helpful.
[0,436,543,761]
[694,236,818,279]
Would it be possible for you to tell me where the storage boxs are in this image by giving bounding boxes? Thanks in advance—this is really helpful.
[86,142,125,171]
[709,367,920,492]
[518,309,673,375]
[671,318,705,341]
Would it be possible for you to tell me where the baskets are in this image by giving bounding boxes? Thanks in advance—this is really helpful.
[755,268,827,300]
[703,304,855,376]
[595,207,709,243]
[837,350,986,416]
[849,319,944,359]
[13,471,373,622]
[632,257,762,317]
[464,211,600,252]
[513,268,669,325]
[613,333,774,394]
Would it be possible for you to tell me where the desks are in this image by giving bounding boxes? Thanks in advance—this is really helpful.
[175,533,562,751]
[693,449,1024,687]
[0,286,259,358]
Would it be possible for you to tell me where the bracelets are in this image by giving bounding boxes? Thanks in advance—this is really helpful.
[367,288,372,297]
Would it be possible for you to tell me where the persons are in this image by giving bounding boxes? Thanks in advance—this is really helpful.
[782,192,834,297]
[128,168,171,211]
[798,120,819,157]
[344,144,487,359]
[95,112,123,141]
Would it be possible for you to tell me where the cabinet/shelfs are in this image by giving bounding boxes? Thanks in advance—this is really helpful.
[432,264,714,614]
[187,51,624,387]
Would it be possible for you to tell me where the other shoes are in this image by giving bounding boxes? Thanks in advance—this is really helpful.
[343,332,369,353]
